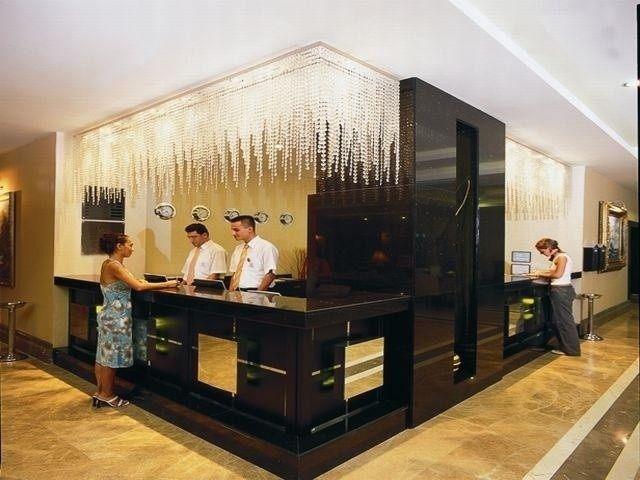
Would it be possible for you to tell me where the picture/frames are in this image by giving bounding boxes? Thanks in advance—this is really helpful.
[597,200,629,274]
[0,191,15,293]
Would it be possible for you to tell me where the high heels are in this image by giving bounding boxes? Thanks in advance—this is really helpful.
[551,349,569,355]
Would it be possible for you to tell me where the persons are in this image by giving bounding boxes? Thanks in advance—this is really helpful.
[178,223,229,289]
[229,215,280,292]
[93,232,181,409]
[530,238,581,358]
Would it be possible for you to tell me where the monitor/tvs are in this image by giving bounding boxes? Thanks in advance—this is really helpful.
[144,274,168,282]
[193,279,226,289]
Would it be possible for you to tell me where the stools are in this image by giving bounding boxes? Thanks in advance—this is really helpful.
[0,300,29,363]
[582,292,604,342]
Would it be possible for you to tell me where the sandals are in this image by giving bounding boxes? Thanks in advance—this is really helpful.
[98,396,129,410]
[90,393,106,407]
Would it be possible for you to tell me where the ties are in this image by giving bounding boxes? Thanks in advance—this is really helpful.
[187,247,201,286]
[229,245,249,291]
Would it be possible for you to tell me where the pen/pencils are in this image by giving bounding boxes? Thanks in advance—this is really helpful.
[175,275,178,282]
